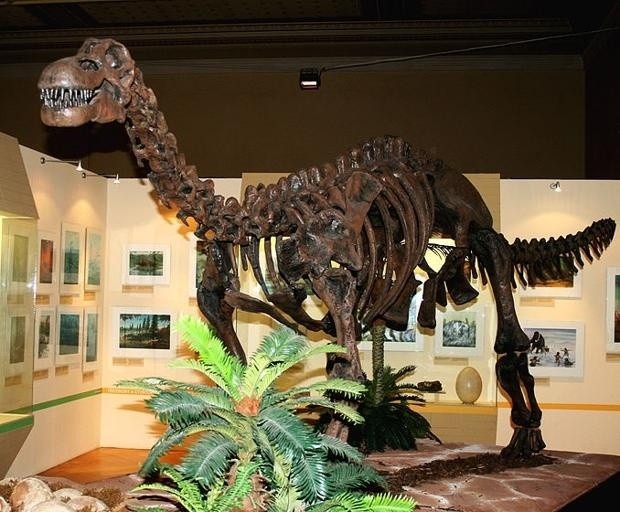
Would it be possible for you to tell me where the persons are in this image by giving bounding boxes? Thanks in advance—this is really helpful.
[560,348,573,359]
[529,356,541,367]
[554,351,563,366]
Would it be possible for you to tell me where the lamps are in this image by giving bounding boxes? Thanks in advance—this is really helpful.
[550,181,561,191]
[299,26,620,89]
[41,156,83,172]
[82,172,120,184]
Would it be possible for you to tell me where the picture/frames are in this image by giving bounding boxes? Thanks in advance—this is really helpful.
[518,251,581,298]
[113,307,176,359]
[4,305,100,380]
[122,242,170,285]
[441,257,478,293]
[256,245,323,306]
[605,266,620,355]
[355,275,424,353]
[5,221,103,296]
[188,233,210,298]
[520,320,584,379]
[433,302,484,357]
